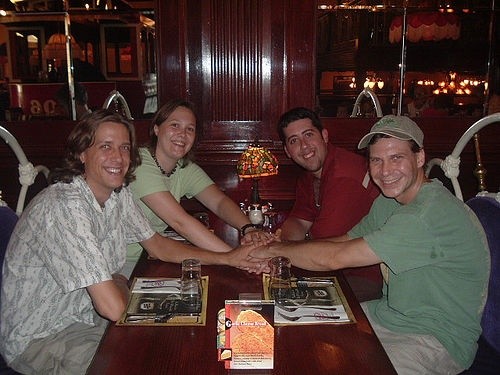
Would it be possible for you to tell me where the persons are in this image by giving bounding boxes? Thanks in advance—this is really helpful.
[0,97,492,375]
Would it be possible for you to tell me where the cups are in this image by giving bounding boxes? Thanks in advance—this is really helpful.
[193,213,209,229]
[268,256,293,302]
[181,257,202,309]
[263,210,279,234]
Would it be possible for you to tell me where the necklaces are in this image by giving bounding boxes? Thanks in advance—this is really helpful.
[152,154,179,177]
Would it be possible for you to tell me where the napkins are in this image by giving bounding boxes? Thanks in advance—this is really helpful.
[273,304,350,323]
[131,278,199,294]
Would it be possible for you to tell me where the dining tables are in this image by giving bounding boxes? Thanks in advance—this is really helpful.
[84,212,399,375]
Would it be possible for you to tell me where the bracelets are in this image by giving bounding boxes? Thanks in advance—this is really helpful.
[240,223,255,236]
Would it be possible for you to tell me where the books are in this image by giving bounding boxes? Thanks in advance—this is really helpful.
[224,300,275,370]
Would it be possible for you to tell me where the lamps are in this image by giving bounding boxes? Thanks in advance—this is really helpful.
[236,136,279,226]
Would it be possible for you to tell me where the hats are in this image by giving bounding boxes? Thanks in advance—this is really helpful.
[358,116,424,151]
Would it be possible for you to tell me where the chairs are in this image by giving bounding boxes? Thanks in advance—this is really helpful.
[444,196,500,375]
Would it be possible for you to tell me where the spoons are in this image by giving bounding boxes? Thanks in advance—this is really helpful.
[127,311,178,322]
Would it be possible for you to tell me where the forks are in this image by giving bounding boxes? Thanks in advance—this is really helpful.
[275,302,337,312]
[277,312,340,322]
[141,280,195,292]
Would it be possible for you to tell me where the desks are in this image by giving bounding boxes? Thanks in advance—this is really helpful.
[320,92,399,118]
